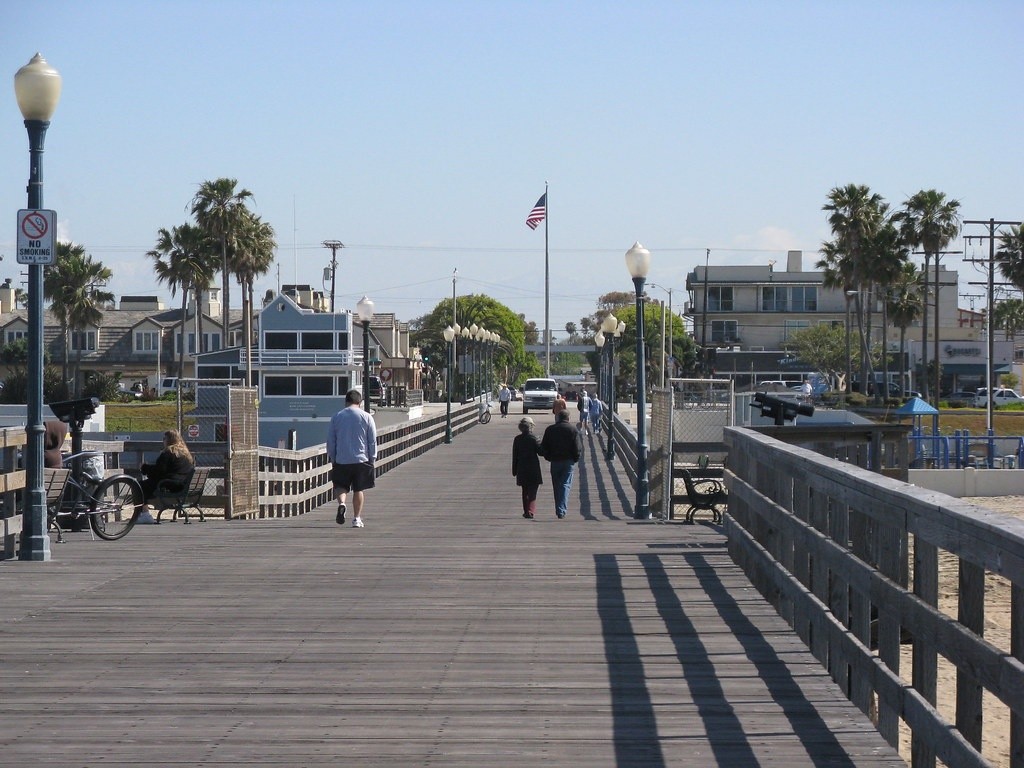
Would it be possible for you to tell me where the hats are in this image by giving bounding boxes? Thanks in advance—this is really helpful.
[522,417,536,428]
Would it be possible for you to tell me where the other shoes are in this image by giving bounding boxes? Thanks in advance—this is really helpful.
[523,513,533,518]
[134,513,155,524]
[337,504,347,525]
[352,519,365,527]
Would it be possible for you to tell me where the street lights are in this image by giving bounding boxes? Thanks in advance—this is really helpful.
[453,322,502,407]
[13,49,61,560]
[442,324,456,444]
[847,290,941,411]
[624,240,651,518]
[355,295,375,415]
[593,312,627,460]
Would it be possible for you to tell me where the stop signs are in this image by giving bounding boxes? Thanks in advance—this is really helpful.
[188,425,199,437]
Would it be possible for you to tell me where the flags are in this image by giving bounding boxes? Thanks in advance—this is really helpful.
[526,193,546,230]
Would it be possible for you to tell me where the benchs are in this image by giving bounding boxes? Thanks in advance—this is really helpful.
[44,468,73,546]
[679,468,727,525]
[150,467,211,524]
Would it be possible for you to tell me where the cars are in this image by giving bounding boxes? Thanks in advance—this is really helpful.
[940,387,1024,409]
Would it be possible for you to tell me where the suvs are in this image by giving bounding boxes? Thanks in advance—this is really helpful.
[847,381,923,400]
[362,376,388,406]
[518,377,559,413]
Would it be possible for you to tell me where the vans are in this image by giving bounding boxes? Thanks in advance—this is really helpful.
[161,377,195,398]
[758,380,804,390]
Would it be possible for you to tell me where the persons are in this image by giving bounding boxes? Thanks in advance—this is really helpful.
[43,422,66,469]
[512,418,543,519]
[540,410,581,519]
[800,379,812,395]
[591,393,603,434]
[553,394,567,423]
[565,384,578,401]
[135,429,195,525]
[579,391,593,436]
[326,390,377,528]
[499,384,511,418]
